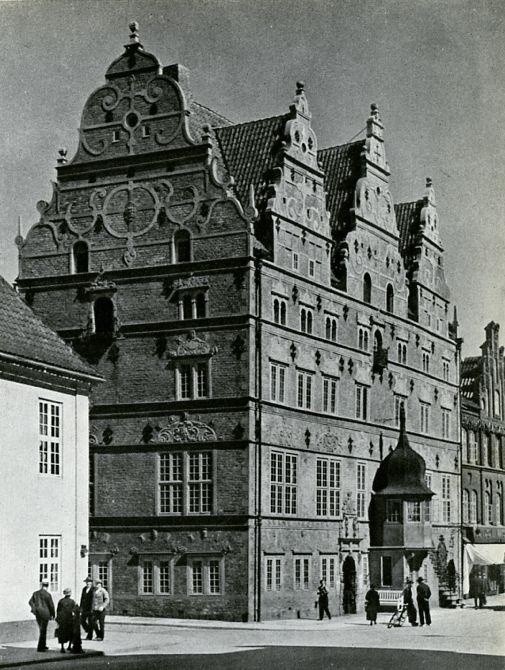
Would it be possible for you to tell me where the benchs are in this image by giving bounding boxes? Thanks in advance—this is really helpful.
[375,589,408,610]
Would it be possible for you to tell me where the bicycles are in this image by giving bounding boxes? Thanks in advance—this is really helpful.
[386,600,410,628]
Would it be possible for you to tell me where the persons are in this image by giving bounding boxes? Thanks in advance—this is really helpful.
[56,588,76,653]
[470,571,487,610]
[365,584,380,625]
[79,577,95,639]
[89,578,110,641]
[416,577,432,626]
[317,580,331,621]
[403,580,419,627]
[28,579,55,652]
